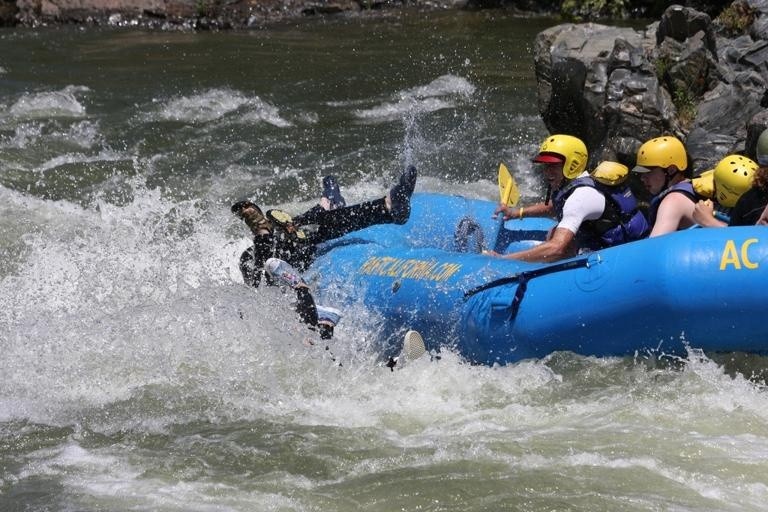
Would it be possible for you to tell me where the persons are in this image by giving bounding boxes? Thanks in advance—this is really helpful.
[631,135,709,240]
[691,153,758,229]
[727,129,768,227]
[227,163,427,375]
[480,129,648,264]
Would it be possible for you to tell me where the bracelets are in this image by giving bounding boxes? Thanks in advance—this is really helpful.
[520,208,524,217]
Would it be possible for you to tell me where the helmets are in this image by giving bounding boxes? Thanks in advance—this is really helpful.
[631,135,688,174]
[532,134,589,179]
[713,154,759,207]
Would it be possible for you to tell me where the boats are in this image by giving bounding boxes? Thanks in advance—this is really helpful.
[304,190,767,369]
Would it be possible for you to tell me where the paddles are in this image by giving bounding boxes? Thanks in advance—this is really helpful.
[488,162,520,251]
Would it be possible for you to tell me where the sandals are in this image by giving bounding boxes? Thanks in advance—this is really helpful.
[230,201,273,235]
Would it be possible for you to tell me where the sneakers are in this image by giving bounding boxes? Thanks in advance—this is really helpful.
[266,209,307,244]
[393,329,426,373]
[390,165,417,226]
[312,306,345,327]
[320,175,346,209]
[264,258,307,289]
